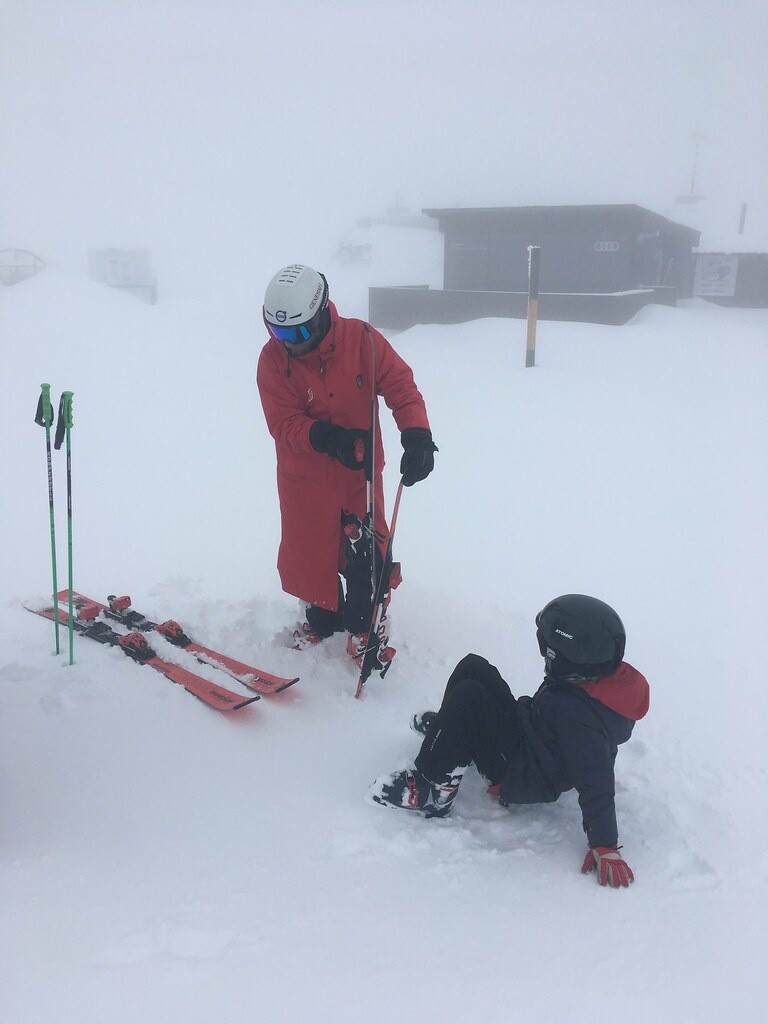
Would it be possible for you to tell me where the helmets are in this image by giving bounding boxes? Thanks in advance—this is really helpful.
[535,594,627,684]
[262,263,329,340]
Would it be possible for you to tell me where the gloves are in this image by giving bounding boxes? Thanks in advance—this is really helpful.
[581,844,636,889]
[309,420,369,469]
[399,428,439,487]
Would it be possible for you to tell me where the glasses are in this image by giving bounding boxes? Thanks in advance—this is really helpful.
[264,322,313,346]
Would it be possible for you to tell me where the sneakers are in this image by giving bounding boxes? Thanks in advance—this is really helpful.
[410,711,438,735]
[292,623,322,651]
[369,769,463,818]
[347,632,369,669]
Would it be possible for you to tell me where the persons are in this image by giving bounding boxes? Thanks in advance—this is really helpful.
[257,263,439,673]
[367,594,649,889]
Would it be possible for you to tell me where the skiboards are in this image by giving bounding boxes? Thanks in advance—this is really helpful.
[20,587,301,712]
[353,322,414,700]
